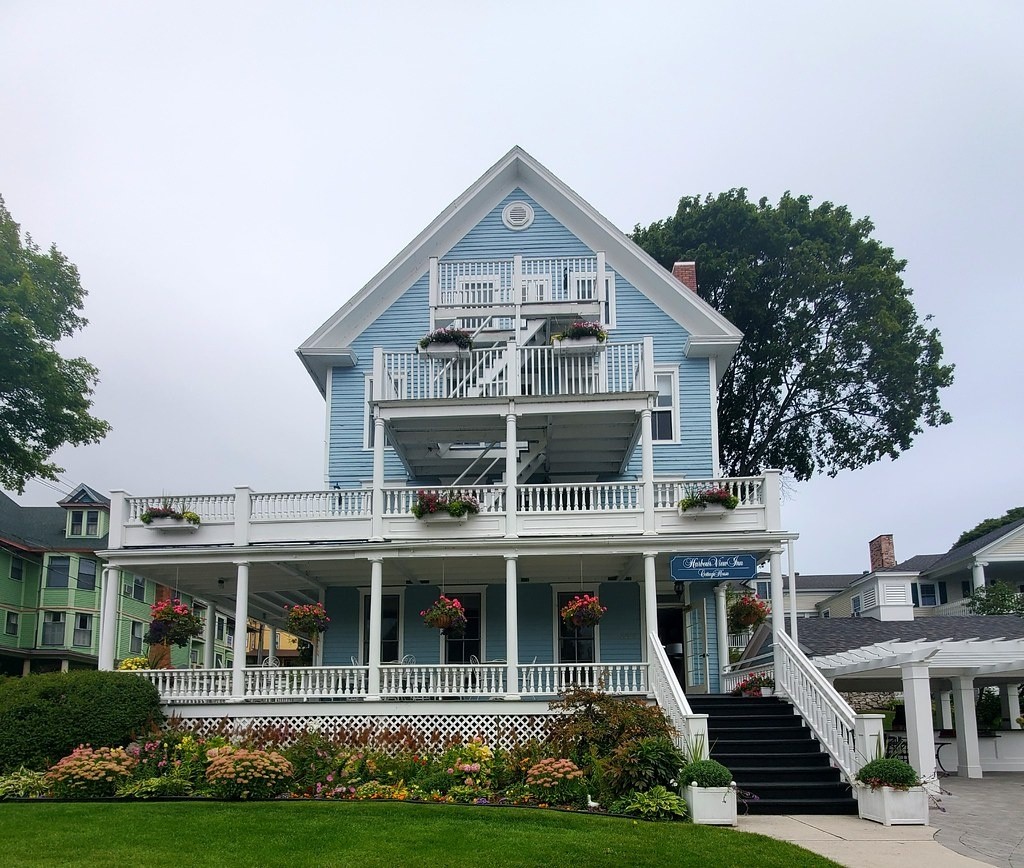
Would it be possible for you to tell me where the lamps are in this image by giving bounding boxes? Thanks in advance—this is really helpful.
[674,581,684,597]
[564,267,570,289]
[334,482,343,505]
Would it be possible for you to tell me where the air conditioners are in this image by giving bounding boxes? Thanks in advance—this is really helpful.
[125,585,132,593]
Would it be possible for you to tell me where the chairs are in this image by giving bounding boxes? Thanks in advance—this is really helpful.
[215,656,280,703]
[470,655,538,691]
[884,735,909,764]
[351,654,416,700]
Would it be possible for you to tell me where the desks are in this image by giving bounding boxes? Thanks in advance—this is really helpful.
[890,740,952,778]
[482,661,507,663]
[366,662,402,700]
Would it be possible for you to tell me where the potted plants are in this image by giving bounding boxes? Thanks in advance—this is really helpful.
[854,758,929,826]
[680,759,738,827]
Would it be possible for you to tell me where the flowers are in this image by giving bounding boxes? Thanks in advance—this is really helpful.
[409,489,480,519]
[139,488,201,525]
[417,596,467,635]
[416,327,474,356]
[677,485,740,512]
[119,598,207,682]
[560,595,607,629]
[550,312,609,343]
[282,603,333,637]
[722,582,775,693]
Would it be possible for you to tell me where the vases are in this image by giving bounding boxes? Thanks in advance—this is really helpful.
[742,687,773,697]
[141,516,199,532]
[413,509,468,522]
[741,615,757,625]
[553,336,606,354]
[417,341,472,358]
[433,615,451,628]
[678,502,732,517]
